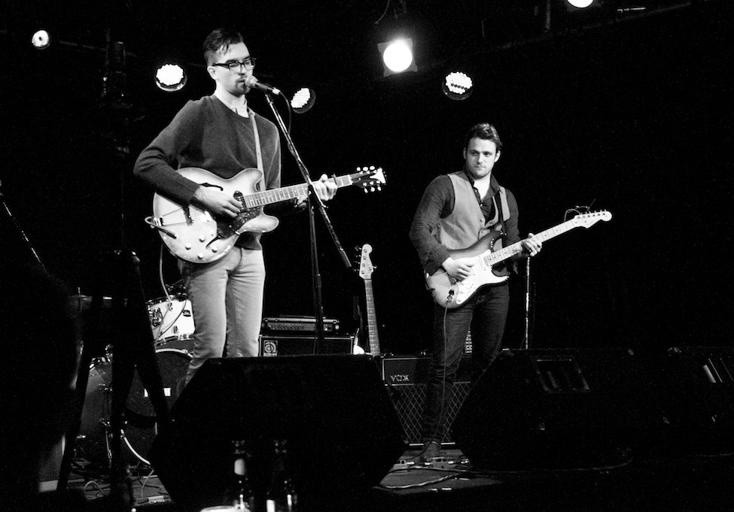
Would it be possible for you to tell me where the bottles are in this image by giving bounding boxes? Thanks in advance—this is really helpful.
[264,439,299,512]
[223,439,258,512]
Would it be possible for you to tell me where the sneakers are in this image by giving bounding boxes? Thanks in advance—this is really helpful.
[421,442,443,462]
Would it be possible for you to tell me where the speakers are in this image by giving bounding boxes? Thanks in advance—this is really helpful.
[259,336,354,358]
[582,346,734,466]
[145,354,407,506]
[447,347,592,472]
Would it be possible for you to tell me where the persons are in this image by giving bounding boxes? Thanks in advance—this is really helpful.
[133,28,338,388]
[410,122,543,463]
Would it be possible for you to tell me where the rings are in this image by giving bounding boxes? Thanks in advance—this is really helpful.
[537,244,540,249]
[528,248,532,254]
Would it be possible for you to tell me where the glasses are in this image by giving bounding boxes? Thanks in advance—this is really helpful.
[212,57,258,73]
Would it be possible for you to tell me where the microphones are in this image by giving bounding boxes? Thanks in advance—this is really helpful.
[246,75,281,95]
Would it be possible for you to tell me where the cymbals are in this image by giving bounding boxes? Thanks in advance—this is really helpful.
[65,293,128,311]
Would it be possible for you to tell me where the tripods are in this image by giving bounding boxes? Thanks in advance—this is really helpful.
[52,138,172,509]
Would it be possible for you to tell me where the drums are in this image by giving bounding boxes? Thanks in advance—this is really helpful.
[145,296,196,352]
[52,353,115,484]
[118,348,190,467]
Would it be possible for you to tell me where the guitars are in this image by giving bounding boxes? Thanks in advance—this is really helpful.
[152,167,388,265]
[423,210,613,307]
[354,245,389,358]
